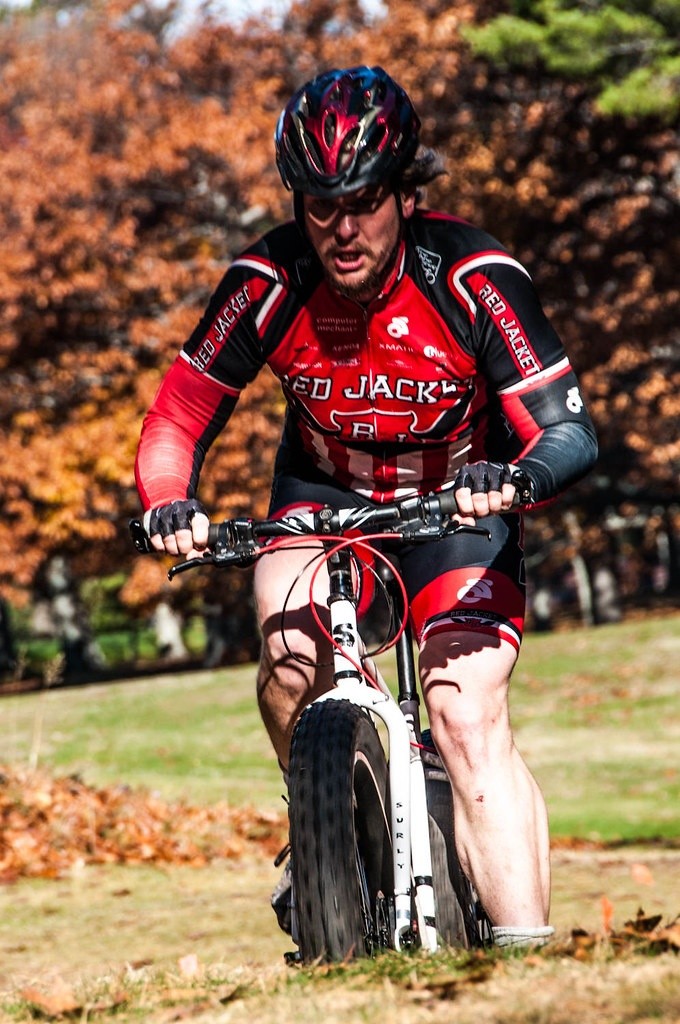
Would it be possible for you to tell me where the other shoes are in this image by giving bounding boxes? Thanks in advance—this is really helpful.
[272,839,293,934]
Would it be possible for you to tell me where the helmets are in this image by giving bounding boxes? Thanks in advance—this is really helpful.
[275,65,422,197]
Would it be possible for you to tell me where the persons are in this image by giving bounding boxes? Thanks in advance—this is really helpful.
[133,64,598,962]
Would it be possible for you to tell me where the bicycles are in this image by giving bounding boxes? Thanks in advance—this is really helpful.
[129,479,519,973]
